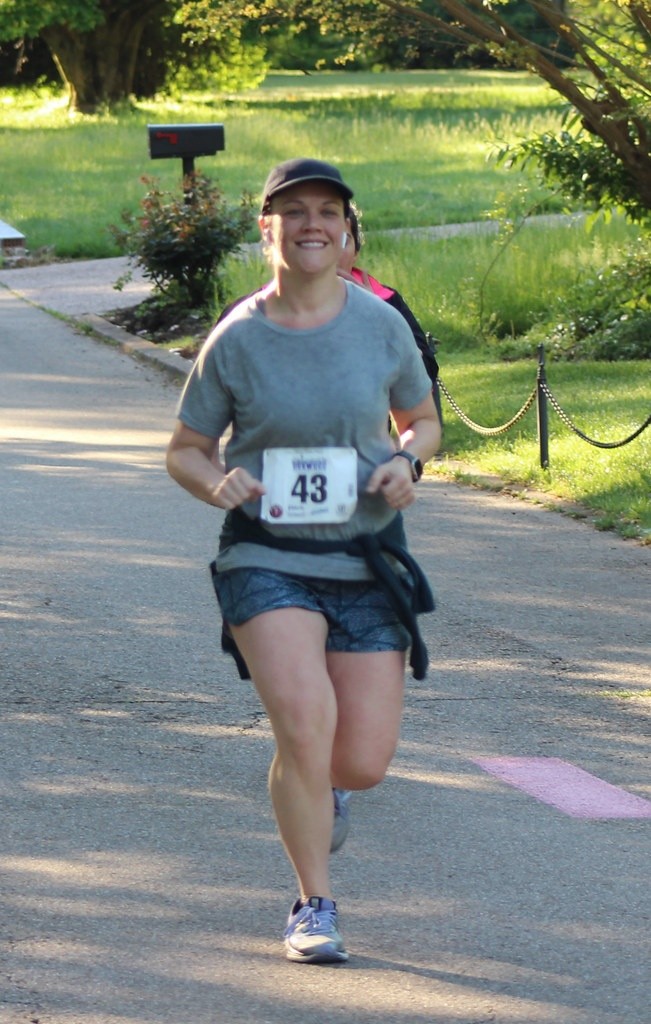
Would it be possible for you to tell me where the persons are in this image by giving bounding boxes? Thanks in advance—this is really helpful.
[166,154,442,962]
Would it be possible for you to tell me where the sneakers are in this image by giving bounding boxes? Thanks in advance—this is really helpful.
[284,896,349,962]
[331,787,352,850]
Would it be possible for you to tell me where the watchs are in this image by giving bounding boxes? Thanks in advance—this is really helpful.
[394,450,423,483]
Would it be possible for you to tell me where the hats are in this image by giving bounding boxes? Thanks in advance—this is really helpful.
[262,158,353,218]
